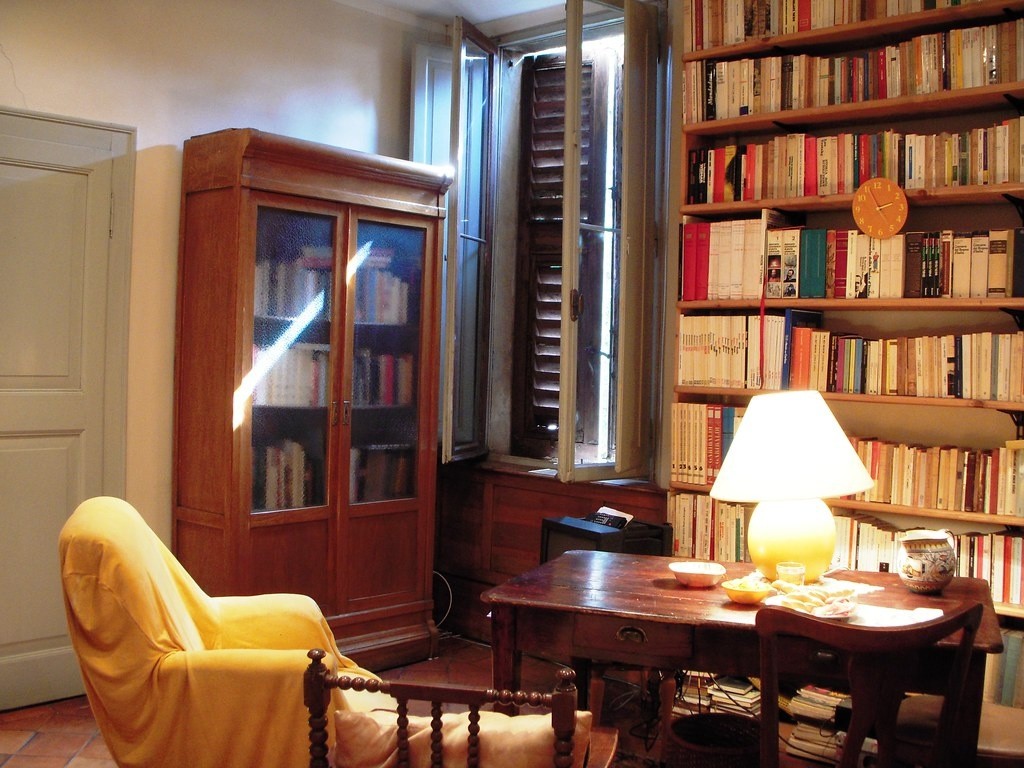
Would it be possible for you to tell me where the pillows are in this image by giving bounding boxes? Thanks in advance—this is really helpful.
[333,706,592,768]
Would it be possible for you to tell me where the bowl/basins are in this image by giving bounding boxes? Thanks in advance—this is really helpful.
[668,562,727,587]
[721,578,771,604]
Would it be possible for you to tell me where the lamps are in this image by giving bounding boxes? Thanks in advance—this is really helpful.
[709,391,875,584]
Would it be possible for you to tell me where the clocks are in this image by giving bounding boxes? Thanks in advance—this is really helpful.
[851,178,907,240]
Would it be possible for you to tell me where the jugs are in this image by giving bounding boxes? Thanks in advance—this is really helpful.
[896,529,956,593]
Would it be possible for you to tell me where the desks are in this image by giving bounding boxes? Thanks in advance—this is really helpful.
[479,549,1005,768]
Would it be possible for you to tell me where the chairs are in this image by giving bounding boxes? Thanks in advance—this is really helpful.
[303,648,620,768]
[54,497,398,768]
[756,602,984,768]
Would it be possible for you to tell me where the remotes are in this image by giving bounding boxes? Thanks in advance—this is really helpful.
[584,512,627,529]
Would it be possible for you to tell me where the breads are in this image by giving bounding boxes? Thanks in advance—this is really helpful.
[771,578,858,614]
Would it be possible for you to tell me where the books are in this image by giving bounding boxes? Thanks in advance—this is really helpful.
[682,0,1024,127]
[254,245,409,324]
[252,428,415,510]
[667,403,1024,606]
[678,118,1024,404]
[671,671,877,768]
[983,626,1024,709]
[254,342,415,405]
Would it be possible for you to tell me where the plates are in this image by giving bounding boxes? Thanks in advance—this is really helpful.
[766,594,859,619]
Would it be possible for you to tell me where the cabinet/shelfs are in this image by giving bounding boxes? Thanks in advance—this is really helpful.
[671,0,1024,760]
[171,127,454,673]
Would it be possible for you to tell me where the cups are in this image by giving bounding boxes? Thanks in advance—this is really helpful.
[775,561,806,587]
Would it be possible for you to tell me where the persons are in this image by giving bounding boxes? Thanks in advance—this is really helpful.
[855,275,866,297]
[787,285,796,296]
[784,269,796,282]
[770,269,780,282]
